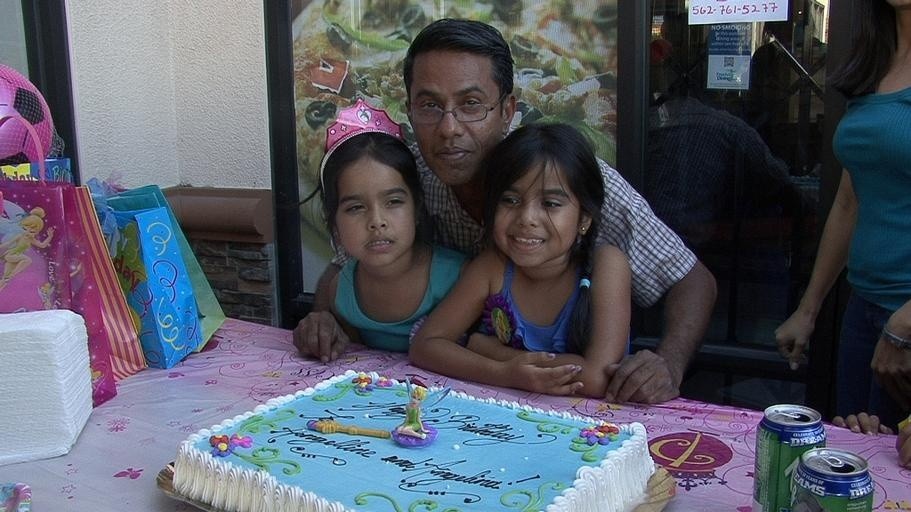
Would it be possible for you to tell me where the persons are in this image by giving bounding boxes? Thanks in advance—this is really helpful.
[319,99,475,354]
[749,0,825,125]
[638,57,811,336]
[834,413,898,436]
[293,19,719,404]
[773,0,911,434]
[894,413,911,471]
[408,127,632,399]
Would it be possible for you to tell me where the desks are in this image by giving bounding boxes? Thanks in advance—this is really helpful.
[0,317,910,512]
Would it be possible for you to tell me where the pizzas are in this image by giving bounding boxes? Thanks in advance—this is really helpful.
[292,1,619,265]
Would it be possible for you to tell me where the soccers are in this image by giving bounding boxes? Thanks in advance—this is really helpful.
[0,63,54,164]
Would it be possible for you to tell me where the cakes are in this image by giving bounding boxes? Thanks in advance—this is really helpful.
[173,368,655,511]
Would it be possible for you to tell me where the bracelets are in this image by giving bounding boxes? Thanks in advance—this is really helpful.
[879,323,910,353]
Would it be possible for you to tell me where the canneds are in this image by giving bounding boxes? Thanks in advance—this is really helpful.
[790,447,873,512]
[752,404,826,511]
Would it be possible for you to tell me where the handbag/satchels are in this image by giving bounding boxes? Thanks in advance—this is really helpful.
[71,185,148,384]
[93,206,203,371]
[0,157,69,183]
[0,180,117,406]
[104,186,226,353]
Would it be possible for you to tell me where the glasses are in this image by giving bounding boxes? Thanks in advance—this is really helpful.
[406,90,506,124]
